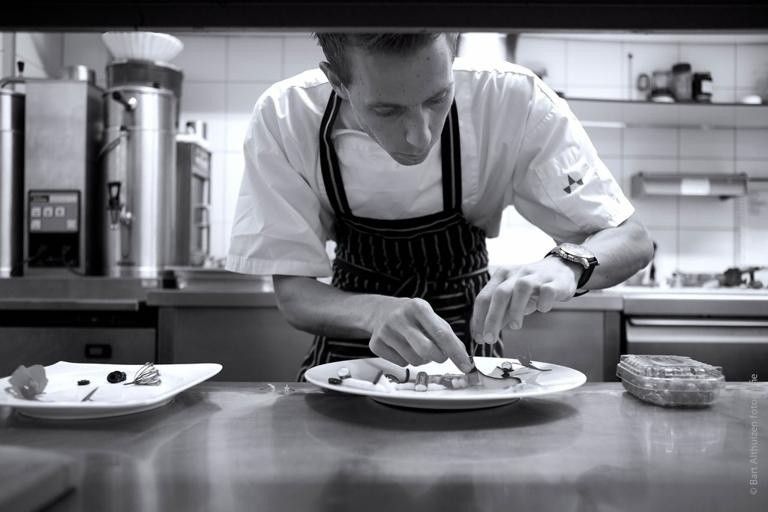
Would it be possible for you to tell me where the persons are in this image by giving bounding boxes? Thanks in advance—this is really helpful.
[220,31,654,384]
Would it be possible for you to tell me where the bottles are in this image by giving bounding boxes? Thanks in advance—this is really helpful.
[670,62,692,103]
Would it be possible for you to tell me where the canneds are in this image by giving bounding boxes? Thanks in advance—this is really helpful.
[693,71,713,103]
[651,70,670,89]
[673,63,694,102]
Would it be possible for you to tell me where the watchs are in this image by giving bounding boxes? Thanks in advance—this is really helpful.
[544,242,600,297]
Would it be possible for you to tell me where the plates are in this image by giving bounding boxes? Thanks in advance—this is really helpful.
[302,355,587,413]
[0,360,224,421]
